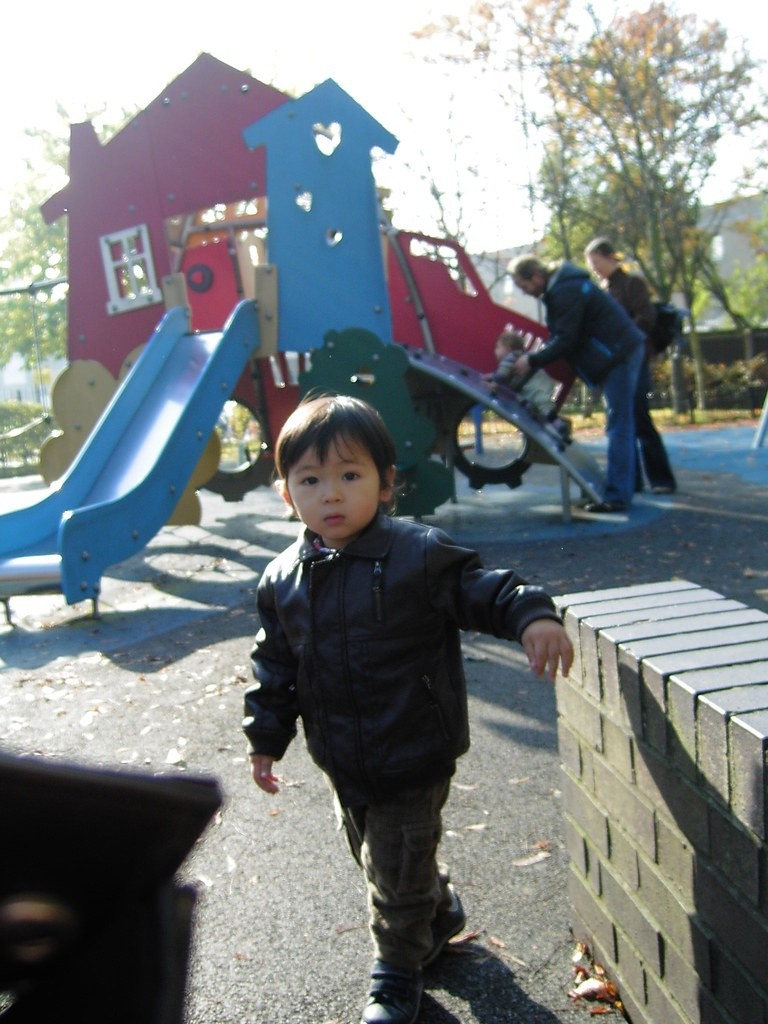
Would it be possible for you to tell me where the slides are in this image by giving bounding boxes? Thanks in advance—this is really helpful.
[0,299,261,606]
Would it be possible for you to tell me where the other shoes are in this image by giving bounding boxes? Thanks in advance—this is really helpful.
[652,477,677,494]
[584,500,625,512]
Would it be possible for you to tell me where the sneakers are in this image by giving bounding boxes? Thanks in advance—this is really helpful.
[360,960,424,1024]
[421,884,466,967]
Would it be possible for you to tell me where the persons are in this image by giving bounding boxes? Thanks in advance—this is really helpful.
[243,395,575,1024]
[586,238,677,494]
[509,254,645,513]
[482,333,573,445]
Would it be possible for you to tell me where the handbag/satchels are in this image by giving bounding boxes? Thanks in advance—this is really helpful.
[649,301,683,351]
